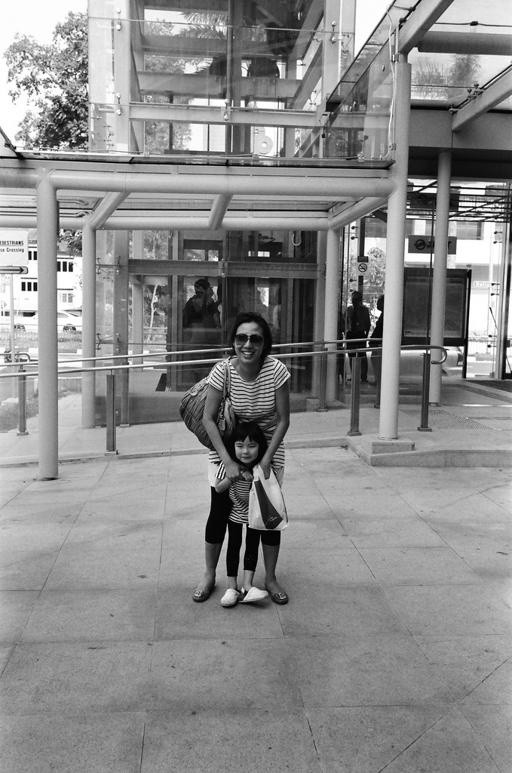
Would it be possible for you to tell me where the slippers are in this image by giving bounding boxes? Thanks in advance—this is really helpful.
[221,587,268,606]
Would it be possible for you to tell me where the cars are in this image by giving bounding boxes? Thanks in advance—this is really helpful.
[10,308,85,336]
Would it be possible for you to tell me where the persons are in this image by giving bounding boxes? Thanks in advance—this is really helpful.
[341,291,371,384]
[256,290,281,330]
[193,312,292,604]
[212,425,275,607]
[368,295,384,408]
[183,278,221,327]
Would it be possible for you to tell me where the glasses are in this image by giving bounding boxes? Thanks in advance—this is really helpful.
[235,334,263,343]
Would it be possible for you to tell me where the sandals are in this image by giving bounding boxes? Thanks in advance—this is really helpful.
[193,589,211,602]
[271,590,288,605]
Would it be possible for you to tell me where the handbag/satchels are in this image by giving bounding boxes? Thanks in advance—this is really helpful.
[179,361,235,451]
[248,463,289,531]
